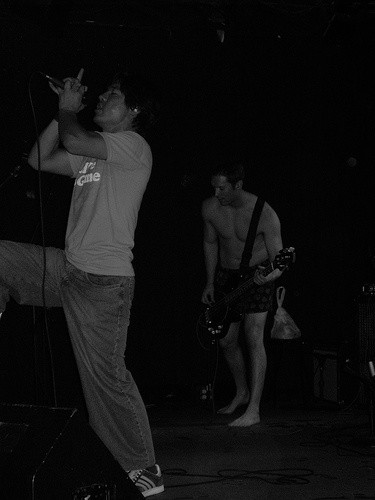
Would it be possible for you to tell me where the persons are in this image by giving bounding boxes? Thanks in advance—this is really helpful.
[0,68,164,500]
[200,161,285,427]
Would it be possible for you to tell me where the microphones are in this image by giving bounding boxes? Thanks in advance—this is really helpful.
[41,73,93,105]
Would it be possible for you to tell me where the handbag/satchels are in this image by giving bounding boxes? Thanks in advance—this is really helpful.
[271,285,301,339]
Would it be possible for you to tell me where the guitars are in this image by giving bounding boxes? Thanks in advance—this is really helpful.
[199,245,296,337]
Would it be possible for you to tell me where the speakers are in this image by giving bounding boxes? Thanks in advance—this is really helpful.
[0,403,118,500]
[312,337,359,405]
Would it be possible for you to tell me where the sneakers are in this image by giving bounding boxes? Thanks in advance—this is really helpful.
[125,463,165,497]
